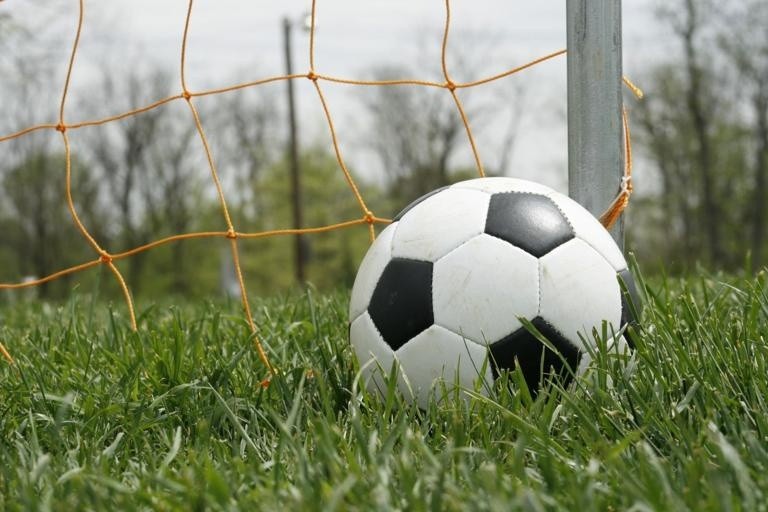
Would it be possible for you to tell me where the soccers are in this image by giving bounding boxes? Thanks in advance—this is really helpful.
[350,176,641,417]
